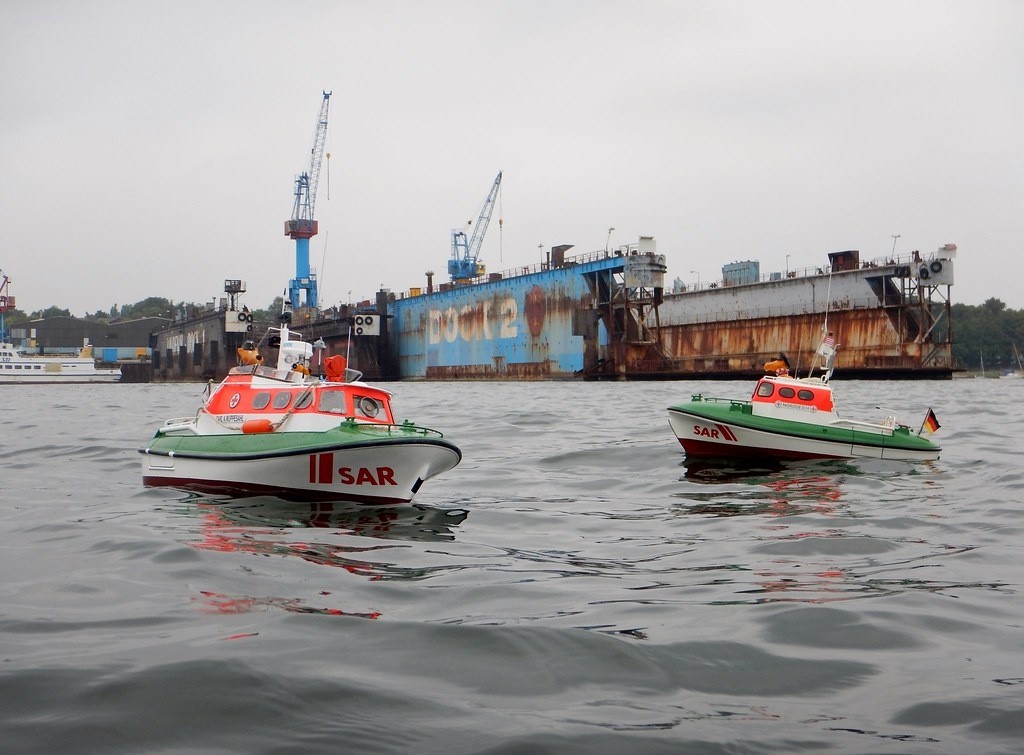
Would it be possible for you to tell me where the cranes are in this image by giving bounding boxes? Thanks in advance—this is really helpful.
[0,269,16,343]
[284,90,333,312]
[448,169,504,289]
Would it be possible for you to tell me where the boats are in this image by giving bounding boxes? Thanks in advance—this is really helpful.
[0,341,123,384]
[665,257,944,461]
[135,311,464,507]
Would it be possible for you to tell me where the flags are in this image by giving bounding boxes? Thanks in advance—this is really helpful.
[923,408,942,434]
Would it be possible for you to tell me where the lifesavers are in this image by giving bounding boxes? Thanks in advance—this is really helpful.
[365,316,373,324]
[356,317,363,325]
[357,328,363,334]
[930,261,942,272]
[894,266,910,277]
[238,313,246,321]
[247,314,253,322]
[920,268,929,279]
[247,325,252,331]
